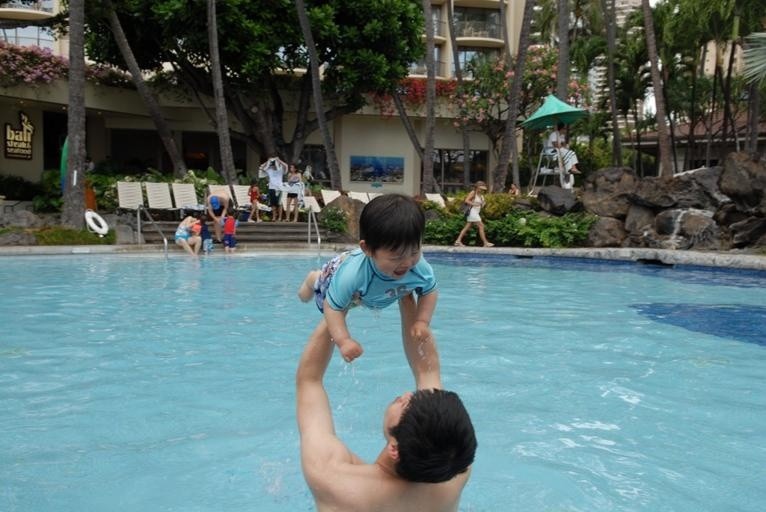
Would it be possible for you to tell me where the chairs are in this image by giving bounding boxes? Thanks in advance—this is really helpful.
[532,128,575,189]
[368,192,384,201]
[301,195,323,214]
[232,184,274,218]
[114,181,150,216]
[207,183,247,218]
[280,196,307,215]
[423,192,447,209]
[170,182,206,218]
[347,191,370,206]
[144,182,186,216]
[319,188,342,206]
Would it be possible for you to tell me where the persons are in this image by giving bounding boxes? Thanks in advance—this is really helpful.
[220,207,237,254]
[509,183,520,195]
[294,192,439,363]
[283,163,302,222]
[174,211,202,257]
[454,180,496,247]
[246,178,262,223]
[197,216,215,257]
[295,287,480,511]
[260,156,289,221]
[547,122,582,175]
[207,189,233,242]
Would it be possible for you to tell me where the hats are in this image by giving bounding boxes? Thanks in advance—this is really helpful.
[474,181,487,191]
[209,195,220,210]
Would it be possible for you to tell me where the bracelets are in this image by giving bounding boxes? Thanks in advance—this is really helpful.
[416,320,430,325]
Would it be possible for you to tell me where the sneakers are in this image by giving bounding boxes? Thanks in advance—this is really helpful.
[454,241,466,247]
[568,168,582,175]
[484,242,494,247]
[247,218,298,224]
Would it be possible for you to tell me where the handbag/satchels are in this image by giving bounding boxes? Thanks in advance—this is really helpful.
[459,202,471,216]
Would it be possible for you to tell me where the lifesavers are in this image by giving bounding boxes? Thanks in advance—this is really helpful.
[84,210,109,236]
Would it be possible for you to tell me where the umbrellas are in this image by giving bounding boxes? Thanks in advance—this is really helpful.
[513,94,588,131]
